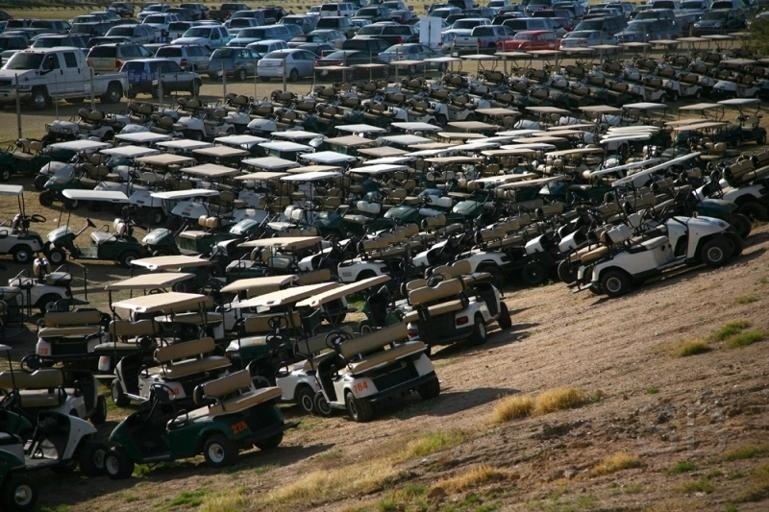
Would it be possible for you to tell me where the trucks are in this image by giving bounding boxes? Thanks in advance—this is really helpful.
[0,47,128,111]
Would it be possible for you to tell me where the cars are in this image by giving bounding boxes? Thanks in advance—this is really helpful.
[207,47,263,81]
[256,48,321,83]
[0,1,768,74]
[314,50,392,83]
[119,58,202,99]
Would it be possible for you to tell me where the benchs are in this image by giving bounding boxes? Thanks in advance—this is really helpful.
[0,57,768,411]
